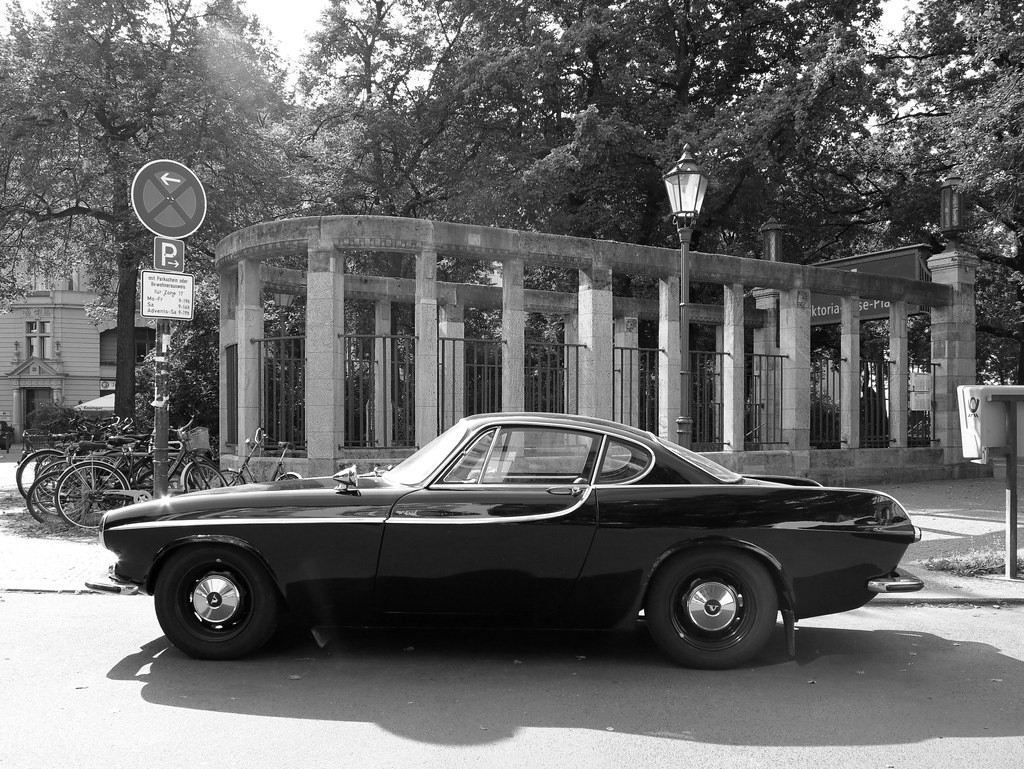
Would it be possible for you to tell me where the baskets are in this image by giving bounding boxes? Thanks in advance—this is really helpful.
[185,427,209,451]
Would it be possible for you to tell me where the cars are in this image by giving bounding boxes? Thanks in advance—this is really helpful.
[85,410,925,671]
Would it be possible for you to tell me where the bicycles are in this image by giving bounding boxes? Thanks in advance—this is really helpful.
[15,415,301,531]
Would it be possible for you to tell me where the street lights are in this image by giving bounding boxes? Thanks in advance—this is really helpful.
[659,141,710,450]
